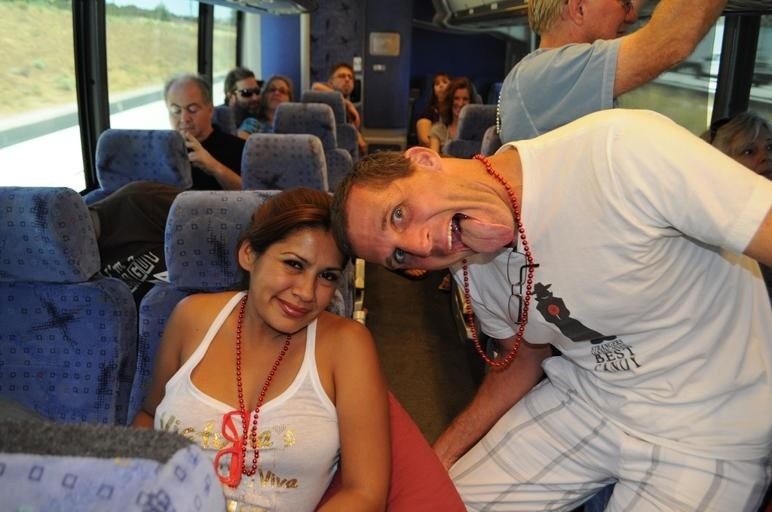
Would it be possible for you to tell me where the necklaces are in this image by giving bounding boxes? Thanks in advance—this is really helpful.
[463,151,531,369]
[236,295,289,477]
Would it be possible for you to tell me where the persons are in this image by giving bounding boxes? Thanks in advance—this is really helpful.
[332,107,771,512]
[122,189,391,511]
[87,181,182,292]
[163,63,366,189]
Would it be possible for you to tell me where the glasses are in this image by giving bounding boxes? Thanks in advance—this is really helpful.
[565,0,634,16]
[266,87,289,94]
[234,87,260,97]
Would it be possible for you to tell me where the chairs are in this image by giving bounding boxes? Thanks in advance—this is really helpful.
[0,86,368,511]
[406,61,512,382]
